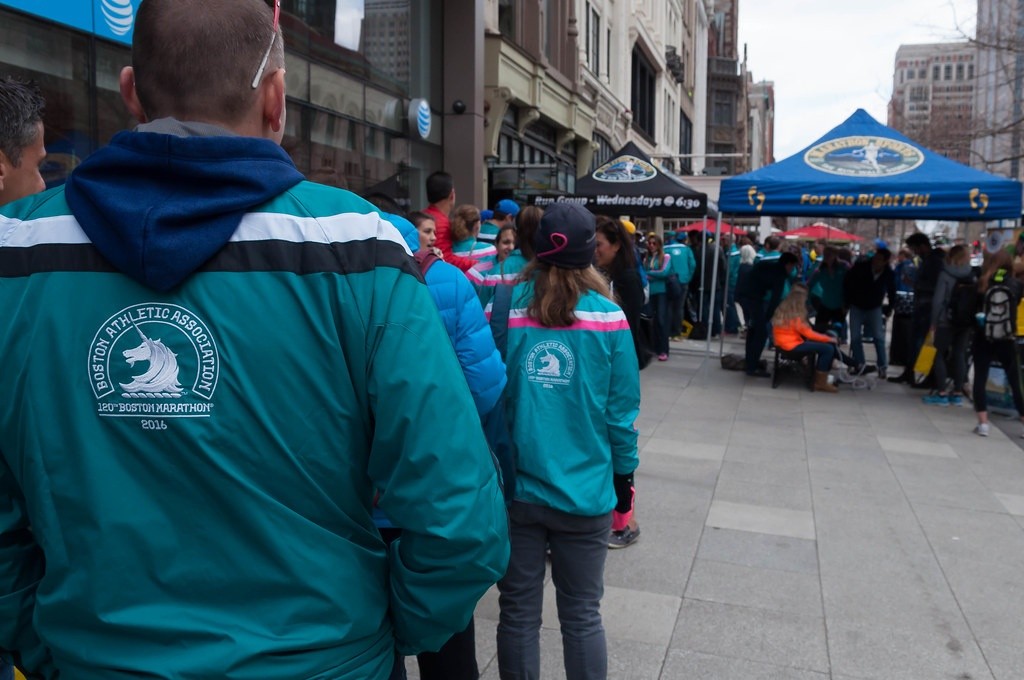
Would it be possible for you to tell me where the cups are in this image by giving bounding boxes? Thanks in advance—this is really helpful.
[828,374,834,384]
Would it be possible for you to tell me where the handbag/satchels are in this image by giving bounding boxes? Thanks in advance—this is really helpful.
[927,354,946,391]
[914,330,938,383]
[662,255,682,300]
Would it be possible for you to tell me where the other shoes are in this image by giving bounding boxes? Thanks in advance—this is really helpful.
[747,368,770,377]
[973,423,989,437]
[879,367,886,378]
[659,352,668,360]
[855,365,875,374]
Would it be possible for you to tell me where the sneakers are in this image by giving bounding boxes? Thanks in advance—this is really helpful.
[608,525,640,548]
[923,394,948,406]
[949,395,962,405]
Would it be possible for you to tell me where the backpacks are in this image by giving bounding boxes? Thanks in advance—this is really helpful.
[983,284,1015,338]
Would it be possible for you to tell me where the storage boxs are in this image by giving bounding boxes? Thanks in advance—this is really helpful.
[968,362,1016,409]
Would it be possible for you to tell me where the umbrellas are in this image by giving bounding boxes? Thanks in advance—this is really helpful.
[676,218,747,234]
[778,222,863,240]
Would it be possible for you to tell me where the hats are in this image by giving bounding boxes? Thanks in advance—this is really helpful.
[534,201,595,268]
[480,210,494,223]
[495,199,520,216]
[677,232,685,241]
[621,218,636,234]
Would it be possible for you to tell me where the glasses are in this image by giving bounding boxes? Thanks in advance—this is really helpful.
[252,0,280,89]
[648,242,657,245]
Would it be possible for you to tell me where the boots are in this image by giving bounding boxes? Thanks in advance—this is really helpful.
[813,371,837,392]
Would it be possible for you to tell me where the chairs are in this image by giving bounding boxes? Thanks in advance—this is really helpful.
[764,300,817,393]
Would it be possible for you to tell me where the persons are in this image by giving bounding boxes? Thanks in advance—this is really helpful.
[0,78,48,206]
[484,202,640,680]
[972,249,1024,438]
[364,190,506,680]
[421,170,479,273]
[0,0,512,680]
[593,217,645,335]
[637,229,978,409]
[449,198,544,304]
[407,209,444,260]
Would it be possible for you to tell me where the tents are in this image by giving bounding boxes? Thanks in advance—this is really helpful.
[704,109,1023,387]
[525,141,718,320]
